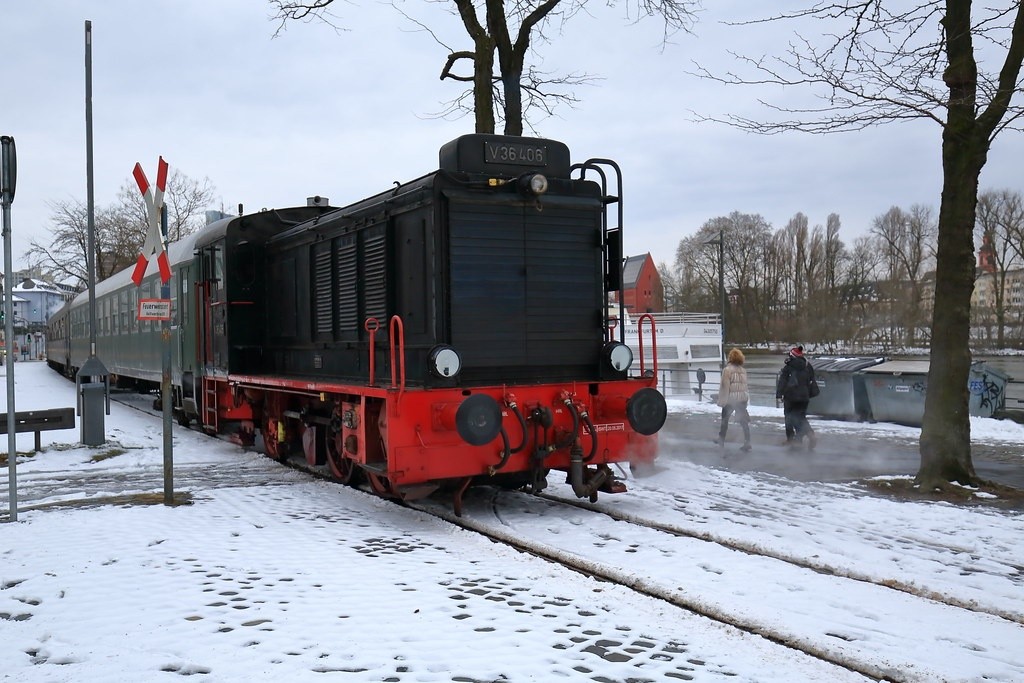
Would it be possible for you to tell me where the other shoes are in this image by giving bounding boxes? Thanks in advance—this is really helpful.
[809,435,817,447]
[713,439,724,446]
[783,440,791,445]
[740,445,753,454]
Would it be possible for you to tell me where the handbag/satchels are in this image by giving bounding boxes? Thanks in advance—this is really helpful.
[809,382,820,398]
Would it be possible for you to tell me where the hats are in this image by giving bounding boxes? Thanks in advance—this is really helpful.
[787,346,803,360]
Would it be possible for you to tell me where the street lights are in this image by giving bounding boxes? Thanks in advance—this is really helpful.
[703,229,725,370]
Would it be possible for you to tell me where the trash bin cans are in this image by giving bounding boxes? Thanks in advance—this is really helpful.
[808,358,884,420]
[861,360,1010,429]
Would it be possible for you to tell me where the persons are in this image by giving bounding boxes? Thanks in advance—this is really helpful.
[713,347,752,449]
[776,346,820,449]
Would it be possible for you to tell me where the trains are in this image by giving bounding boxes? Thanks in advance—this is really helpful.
[40,130,669,521]
